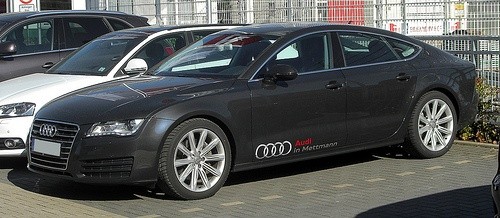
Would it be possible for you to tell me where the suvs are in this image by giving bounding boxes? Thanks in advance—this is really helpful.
[0,9,150,83]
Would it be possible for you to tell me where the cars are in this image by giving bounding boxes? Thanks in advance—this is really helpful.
[0,24,478,201]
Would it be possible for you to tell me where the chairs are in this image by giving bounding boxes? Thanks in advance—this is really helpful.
[144,43,164,69]
[174,36,186,51]
[368,40,395,63]
[253,40,276,69]
